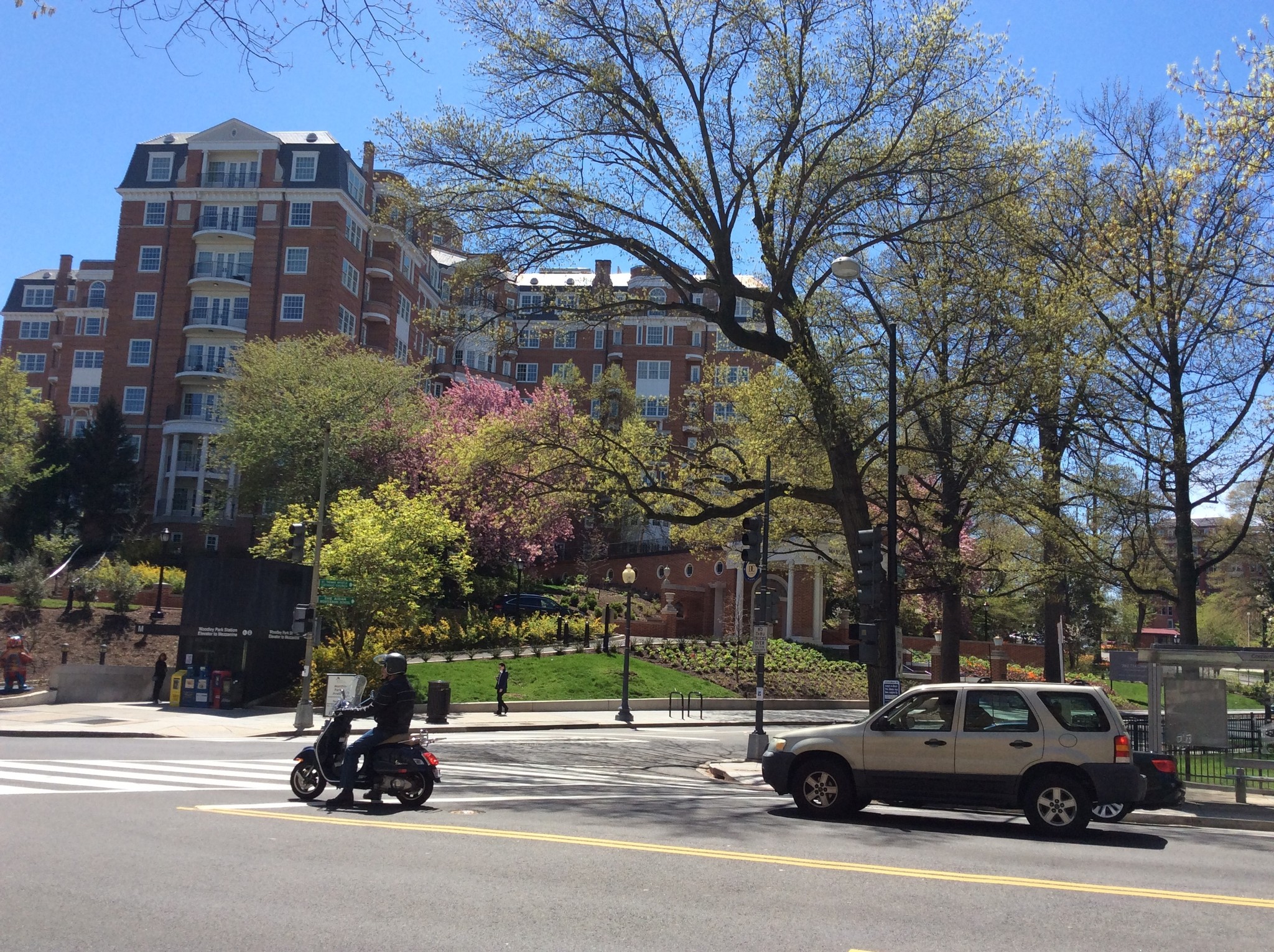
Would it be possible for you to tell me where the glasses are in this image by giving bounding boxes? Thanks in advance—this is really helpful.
[384,664,387,668]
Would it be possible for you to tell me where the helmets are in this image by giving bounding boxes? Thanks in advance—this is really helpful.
[380,653,406,675]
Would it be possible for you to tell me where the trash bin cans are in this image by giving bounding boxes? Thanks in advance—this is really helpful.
[170,665,242,710]
[425,680,451,724]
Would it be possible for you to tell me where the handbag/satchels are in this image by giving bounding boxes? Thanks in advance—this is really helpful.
[153,672,158,681]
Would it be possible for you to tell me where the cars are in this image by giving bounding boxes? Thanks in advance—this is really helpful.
[1009,632,1037,645]
[981,721,1186,815]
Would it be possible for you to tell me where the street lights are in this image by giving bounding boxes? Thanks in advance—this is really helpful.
[151,528,171,617]
[983,601,990,641]
[516,555,526,625]
[615,563,635,720]
[1173,633,1181,673]
[833,254,901,719]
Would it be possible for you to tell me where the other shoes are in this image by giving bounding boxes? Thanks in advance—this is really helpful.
[494,712,501,714]
[157,699,161,703]
[325,792,354,807]
[363,788,382,800]
[505,707,508,713]
[153,700,158,704]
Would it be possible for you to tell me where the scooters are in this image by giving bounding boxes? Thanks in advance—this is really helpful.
[289,690,442,807]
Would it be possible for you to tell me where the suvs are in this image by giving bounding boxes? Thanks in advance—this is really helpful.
[489,592,585,622]
[762,678,1146,842]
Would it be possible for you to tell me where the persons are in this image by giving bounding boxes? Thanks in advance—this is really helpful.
[936,697,956,732]
[493,663,509,714]
[151,653,168,703]
[326,652,414,808]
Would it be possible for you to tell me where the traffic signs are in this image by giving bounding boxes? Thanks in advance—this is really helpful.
[317,579,356,606]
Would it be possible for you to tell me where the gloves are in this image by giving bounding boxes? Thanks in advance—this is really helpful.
[334,708,346,716]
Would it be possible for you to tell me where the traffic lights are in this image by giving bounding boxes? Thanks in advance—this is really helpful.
[286,522,307,562]
[848,622,879,668]
[856,526,883,608]
[741,514,764,567]
[293,607,314,634]
